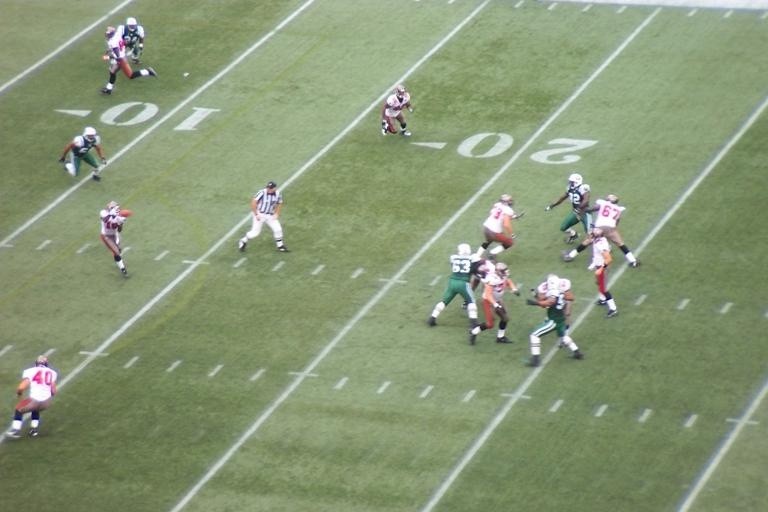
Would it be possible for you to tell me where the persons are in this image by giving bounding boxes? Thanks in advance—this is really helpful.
[60,126,106,181]
[3,353,57,440]
[526,274,582,366]
[474,193,526,259]
[101,200,132,279]
[428,243,519,345]
[544,174,639,320]
[98,26,158,95]
[118,16,144,64]
[238,182,288,255]
[381,84,413,138]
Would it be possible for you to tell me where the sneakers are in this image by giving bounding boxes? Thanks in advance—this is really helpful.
[6,430,21,438]
[28,428,38,437]
[100,88,111,94]
[121,268,128,278]
[427,300,617,366]
[381,123,388,135]
[401,128,411,136]
[278,246,288,252]
[145,66,157,76]
[238,239,246,252]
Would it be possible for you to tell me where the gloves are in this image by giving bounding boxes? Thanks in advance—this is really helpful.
[58,156,65,162]
[110,208,117,216]
[102,157,107,164]
[382,120,387,130]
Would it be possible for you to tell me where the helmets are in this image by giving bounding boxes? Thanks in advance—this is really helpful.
[107,201,119,211]
[456,173,618,290]
[35,355,48,368]
[83,127,96,143]
[395,85,405,98]
[126,17,137,31]
[105,26,115,38]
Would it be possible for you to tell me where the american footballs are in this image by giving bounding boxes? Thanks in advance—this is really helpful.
[117,210,131,217]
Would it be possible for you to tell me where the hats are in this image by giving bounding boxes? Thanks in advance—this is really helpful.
[266,182,276,189]
